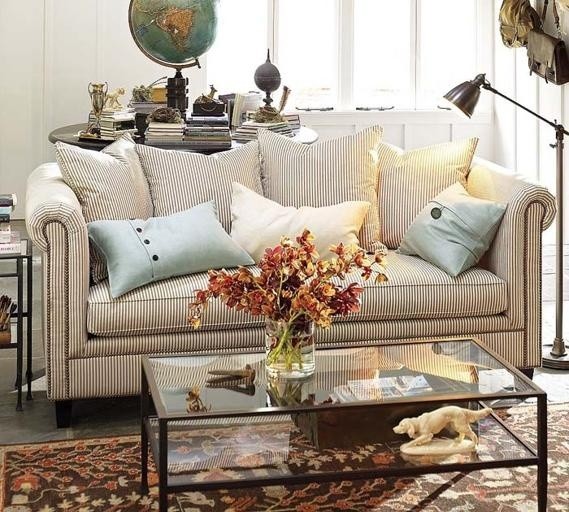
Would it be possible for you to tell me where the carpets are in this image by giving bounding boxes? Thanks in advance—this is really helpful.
[0,402,569,511]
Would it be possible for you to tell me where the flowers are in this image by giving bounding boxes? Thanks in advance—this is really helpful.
[186,227,389,373]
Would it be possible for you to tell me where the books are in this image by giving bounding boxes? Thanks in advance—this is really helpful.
[328,373,435,403]
[0,191,22,256]
[83,85,302,147]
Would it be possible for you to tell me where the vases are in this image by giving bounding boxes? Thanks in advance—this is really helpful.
[264,314,316,381]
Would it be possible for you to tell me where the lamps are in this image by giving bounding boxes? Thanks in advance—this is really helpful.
[442,72,569,371]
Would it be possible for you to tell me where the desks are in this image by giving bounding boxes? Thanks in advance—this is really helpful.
[0,219,33,410]
[47,122,321,156]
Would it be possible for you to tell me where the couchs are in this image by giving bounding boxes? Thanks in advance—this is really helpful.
[24,157,560,428]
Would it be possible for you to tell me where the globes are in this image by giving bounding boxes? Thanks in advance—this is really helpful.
[128,0,219,113]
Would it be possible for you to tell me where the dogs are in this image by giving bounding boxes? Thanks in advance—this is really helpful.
[393,405,494,447]
